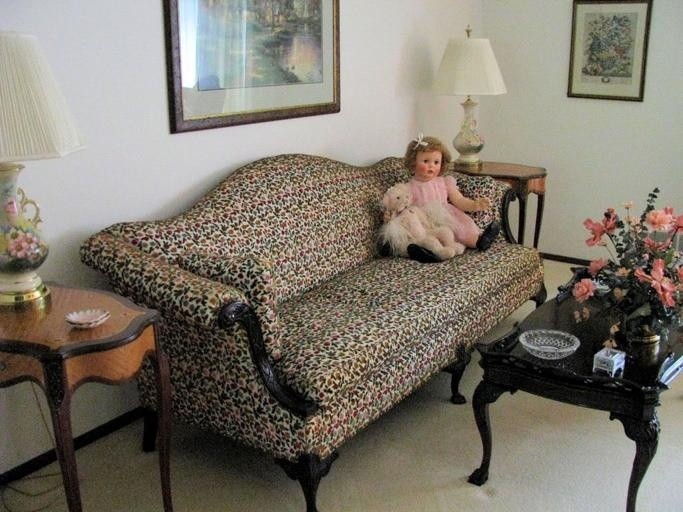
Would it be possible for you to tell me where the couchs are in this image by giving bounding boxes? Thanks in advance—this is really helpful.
[80,150,548,512]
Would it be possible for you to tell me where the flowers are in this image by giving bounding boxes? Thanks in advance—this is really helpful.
[562,178,683,339]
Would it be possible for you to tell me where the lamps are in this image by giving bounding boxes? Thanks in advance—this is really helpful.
[0,30,76,319]
[435,23,509,171]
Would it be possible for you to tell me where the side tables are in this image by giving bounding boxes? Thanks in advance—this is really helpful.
[455,159,546,256]
[0,283,184,511]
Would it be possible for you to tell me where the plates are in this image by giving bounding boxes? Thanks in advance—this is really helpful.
[64,308,109,324]
[64,313,110,331]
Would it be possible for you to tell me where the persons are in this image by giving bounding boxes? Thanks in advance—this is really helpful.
[376,133,502,262]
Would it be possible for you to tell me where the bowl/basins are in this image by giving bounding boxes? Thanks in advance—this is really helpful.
[518,326,582,361]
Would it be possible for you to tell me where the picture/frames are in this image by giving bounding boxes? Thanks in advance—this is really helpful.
[566,0,652,102]
[161,0,341,132]
